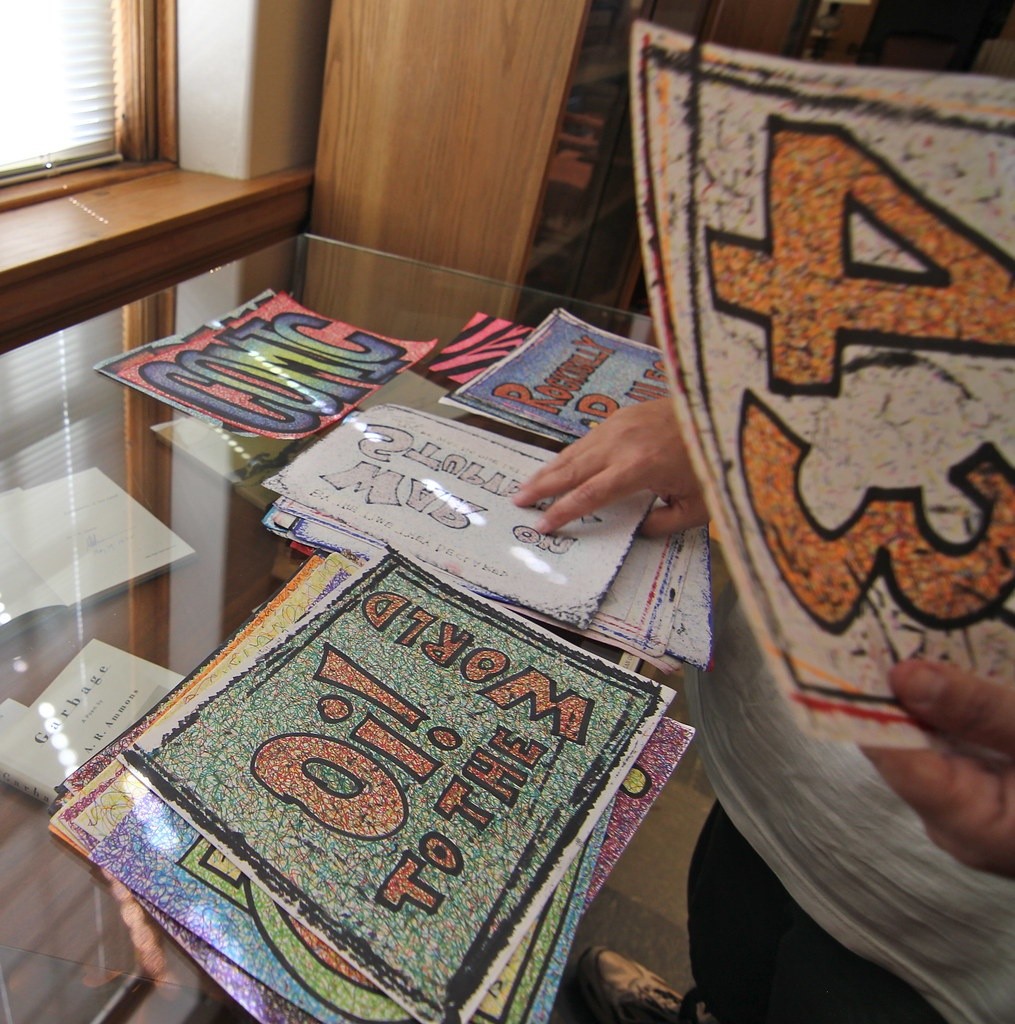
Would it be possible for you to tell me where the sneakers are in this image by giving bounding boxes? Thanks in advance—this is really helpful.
[576,944,685,1024]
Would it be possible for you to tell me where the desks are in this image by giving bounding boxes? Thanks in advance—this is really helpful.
[0,230,676,1024]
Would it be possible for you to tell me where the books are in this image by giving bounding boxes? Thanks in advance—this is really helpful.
[0,638,188,805]
[0,466,200,640]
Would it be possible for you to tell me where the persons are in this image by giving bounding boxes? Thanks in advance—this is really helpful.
[513,392,1015,1024]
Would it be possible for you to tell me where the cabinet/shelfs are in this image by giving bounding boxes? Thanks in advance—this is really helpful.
[307,0,732,308]
